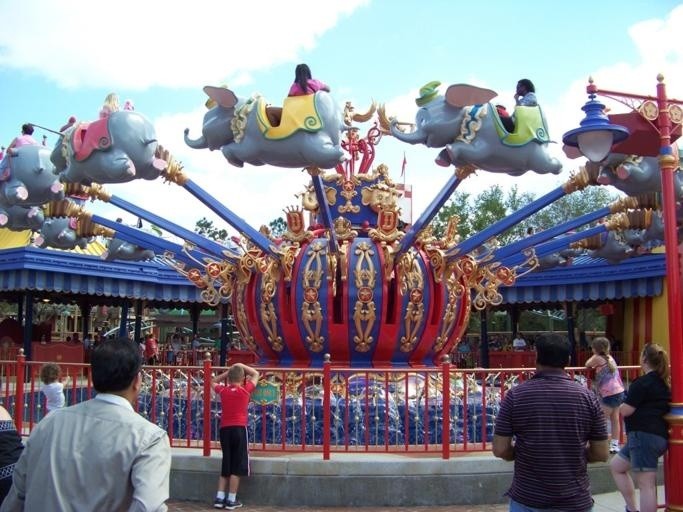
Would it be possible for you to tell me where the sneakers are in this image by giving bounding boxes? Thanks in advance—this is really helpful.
[214,495,244,510]
[608,443,621,454]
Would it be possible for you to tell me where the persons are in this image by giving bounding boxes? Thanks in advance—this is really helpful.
[0,333,171,511]
[492,331,611,511]
[493,77,538,133]
[97,92,120,120]
[584,336,626,453]
[525,225,535,239]
[0,403,26,509]
[208,361,260,509]
[64,316,223,368]
[452,330,538,367]
[608,342,673,512]
[39,361,72,415]
[264,63,331,129]
[3,123,42,153]
